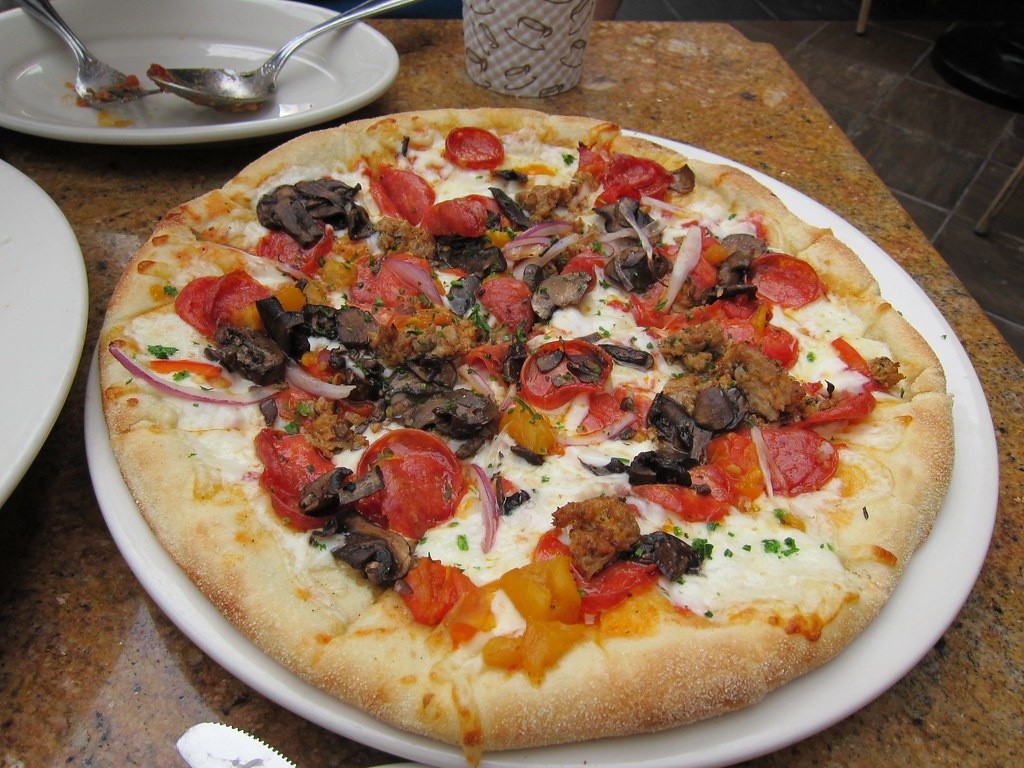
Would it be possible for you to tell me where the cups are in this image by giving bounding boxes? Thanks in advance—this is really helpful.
[451,0,595,97]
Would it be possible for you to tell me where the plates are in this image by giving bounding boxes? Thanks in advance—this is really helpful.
[0,157,90,508]
[0,0,402,145]
[84,125,999,768]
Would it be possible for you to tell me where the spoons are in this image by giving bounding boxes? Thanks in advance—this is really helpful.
[146,0,417,77]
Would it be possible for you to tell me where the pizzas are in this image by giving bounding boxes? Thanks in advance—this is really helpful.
[99,106,956,755]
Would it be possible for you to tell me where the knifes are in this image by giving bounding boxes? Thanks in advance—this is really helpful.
[174,721,302,768]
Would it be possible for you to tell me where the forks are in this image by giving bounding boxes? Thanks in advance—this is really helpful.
[16,0,163,109]
